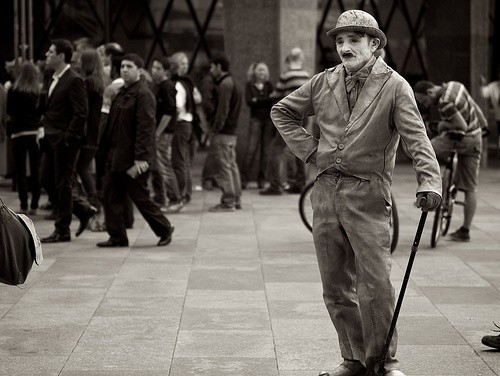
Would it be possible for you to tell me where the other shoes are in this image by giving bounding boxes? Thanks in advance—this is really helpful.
[481,334,500,349]
[284,186,302,193]
[449,230,470,241]
[319,365,355,376]
[260,188,281,195]
[208,203,235,212]
[384,369,405,376]
[159,201,186,213]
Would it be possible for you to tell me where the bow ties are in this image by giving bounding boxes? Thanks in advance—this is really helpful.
[345,72,367,93]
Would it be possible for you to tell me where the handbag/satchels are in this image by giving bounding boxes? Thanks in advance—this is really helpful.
[0,199,43,286]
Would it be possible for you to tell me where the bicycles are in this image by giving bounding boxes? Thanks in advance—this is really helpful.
[429,127,459,246]
[297,177,398,252]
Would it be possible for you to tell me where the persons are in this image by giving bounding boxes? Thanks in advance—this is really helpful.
[415,79,482,241]
[1,38,241,247]
[270,10,443,376]
[243,61,275,189]
[260,48,311,196]
[482,331,500,351]
[0,203,42,286]
[479,74,500,157]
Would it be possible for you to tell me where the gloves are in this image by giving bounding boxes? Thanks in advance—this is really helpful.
[415,192,441,212]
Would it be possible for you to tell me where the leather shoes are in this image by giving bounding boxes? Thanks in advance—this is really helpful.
[40,230,71,243]
[96,237,128,248]
[158,225,174,246]
[75,206,97,237]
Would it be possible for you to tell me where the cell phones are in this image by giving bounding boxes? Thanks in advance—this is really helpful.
[126,165,139,178]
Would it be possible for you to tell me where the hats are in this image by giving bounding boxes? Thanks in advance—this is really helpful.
[326,10,387,49]
